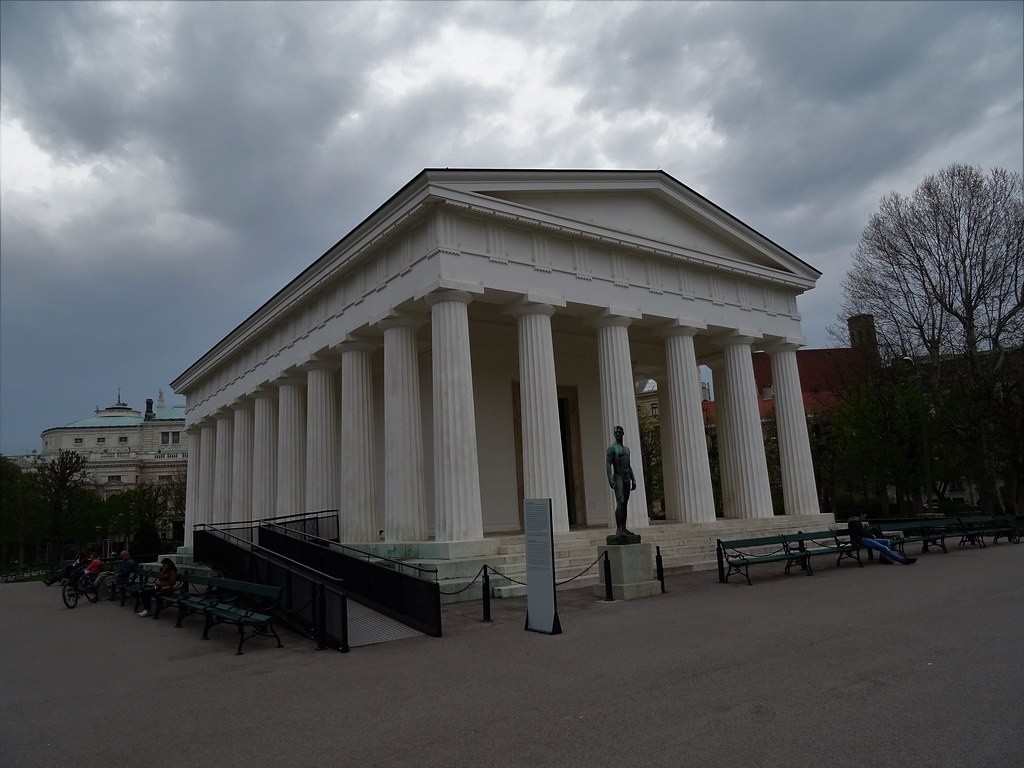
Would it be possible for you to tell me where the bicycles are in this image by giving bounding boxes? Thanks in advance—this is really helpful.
[61,568,99,609]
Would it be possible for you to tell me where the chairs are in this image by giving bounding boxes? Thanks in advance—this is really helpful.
[110,568,282,656]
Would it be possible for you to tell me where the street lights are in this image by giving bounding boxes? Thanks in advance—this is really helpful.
[902,356,932,512]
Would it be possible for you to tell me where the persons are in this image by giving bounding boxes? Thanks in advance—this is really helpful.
[78,552,102,576]
[848,511,917,565]
[606,426,636,536]
[138,558,178,617]
[94,551,134,601]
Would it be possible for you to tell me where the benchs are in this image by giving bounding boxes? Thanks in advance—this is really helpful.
[718,515,1024,586]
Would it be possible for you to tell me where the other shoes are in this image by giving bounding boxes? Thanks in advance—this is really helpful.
[88,585,95,591]
[138,609,152,617]
[42,579,50,586]
